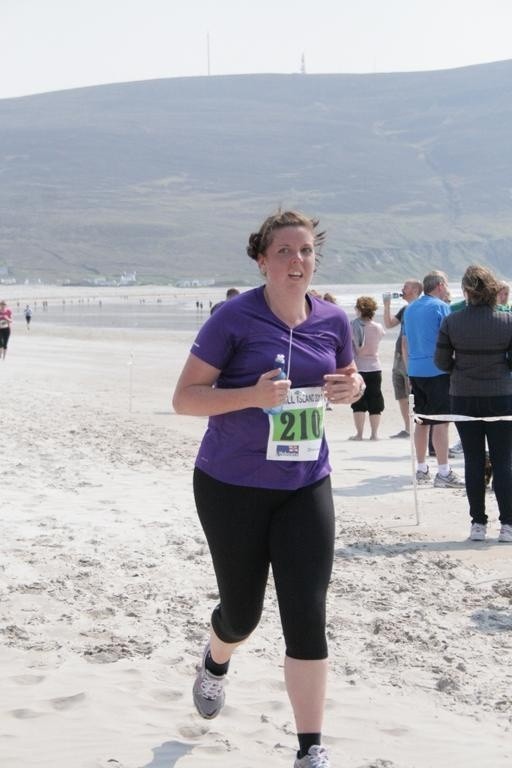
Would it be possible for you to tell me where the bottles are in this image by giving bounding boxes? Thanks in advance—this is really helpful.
[259,354,290,416]
[381,292,403,300]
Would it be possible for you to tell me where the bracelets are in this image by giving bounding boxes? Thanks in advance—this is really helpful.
[353,379,365,399]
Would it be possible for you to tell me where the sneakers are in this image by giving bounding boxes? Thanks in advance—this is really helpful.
[434,471,465,488]
[416,465,432,484]
[470,521,486,540]
[192,644,227,720]
[293,745,329,768]
[498,524,512,542]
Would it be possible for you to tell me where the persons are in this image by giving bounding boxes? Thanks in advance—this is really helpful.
[24,305,31,330]
[451,297,512,454]
[401,269,466,489]
[209,287,338,312]
[0,301,13,359]
[432,264,511,544]
[172,204,368,768]
[382,278,424,439]
[496,281,510,305]
[428,280,454,459]
[347,296,384,441]
